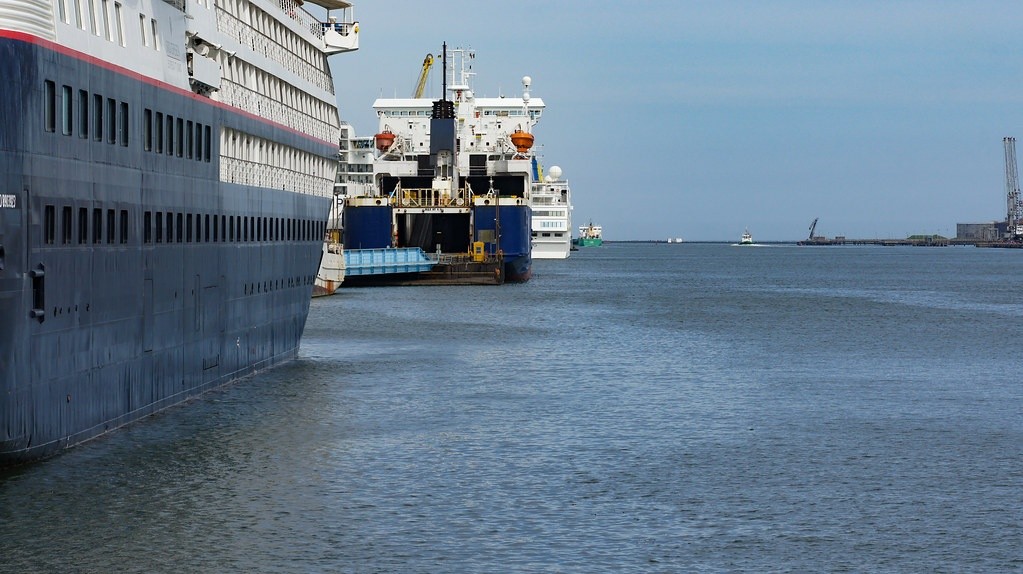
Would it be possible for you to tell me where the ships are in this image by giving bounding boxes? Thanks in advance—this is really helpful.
[0,0,361,466]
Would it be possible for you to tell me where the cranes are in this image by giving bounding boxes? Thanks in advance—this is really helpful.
[809,216,821,239]
[1002,136,1023,241]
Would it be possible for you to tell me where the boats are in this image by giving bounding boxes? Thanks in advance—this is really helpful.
[326,37,575,282]
[740,230,752,244]
[509,131,536,153]
[375,131,396,150]
[571,223,604,248]
